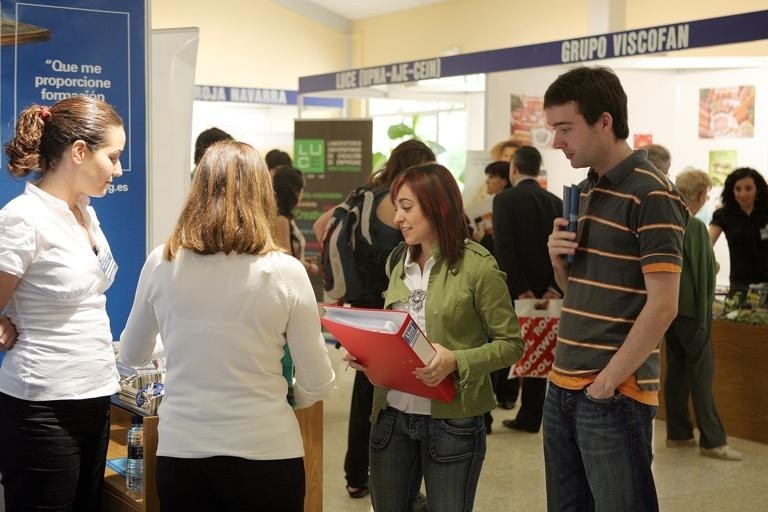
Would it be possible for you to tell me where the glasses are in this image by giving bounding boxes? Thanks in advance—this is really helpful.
[703,191,710,201]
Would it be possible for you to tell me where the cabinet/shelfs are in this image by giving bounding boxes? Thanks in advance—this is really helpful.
[100,399,322,512]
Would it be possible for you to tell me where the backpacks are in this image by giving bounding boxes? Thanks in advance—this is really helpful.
[319,185,392,299]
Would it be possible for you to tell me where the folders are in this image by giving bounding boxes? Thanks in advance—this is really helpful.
[320,303,456,404]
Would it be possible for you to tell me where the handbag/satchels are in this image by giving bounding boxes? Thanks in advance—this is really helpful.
[506,299,563,380]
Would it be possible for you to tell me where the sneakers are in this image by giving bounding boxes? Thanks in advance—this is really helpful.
[699,446,742,460]
[666,437,699,448]
[346,484,368,498]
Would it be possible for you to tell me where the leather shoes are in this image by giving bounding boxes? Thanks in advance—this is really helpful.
[498,400,515,409]
[502,420,538,433]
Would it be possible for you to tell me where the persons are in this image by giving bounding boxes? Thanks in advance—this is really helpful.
[264,148,292,185]
[539,65,692,512]
[269,164,321,407]
[639,143,672,178]
[311,126,565,511]
[119,138,333,512]
[0,92,130,511]
[707,166,767,314]
[661,169,746,461]
[191,125,236,180]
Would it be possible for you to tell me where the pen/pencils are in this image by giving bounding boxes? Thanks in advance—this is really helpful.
[344,359,351,372]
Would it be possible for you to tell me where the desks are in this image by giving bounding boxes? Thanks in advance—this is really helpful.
[654,316,768,445]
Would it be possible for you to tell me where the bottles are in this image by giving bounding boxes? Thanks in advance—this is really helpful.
[125,415,144,493]
